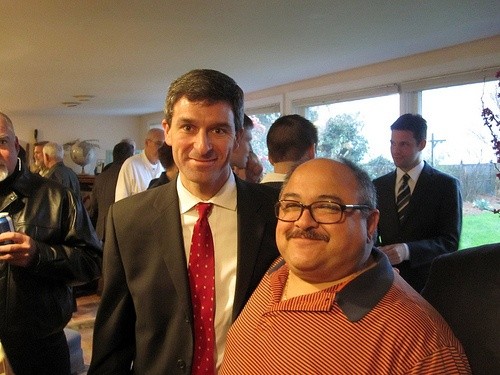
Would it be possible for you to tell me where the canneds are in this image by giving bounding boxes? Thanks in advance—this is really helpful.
[0,212,15,245]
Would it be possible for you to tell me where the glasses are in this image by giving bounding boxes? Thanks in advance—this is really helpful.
[273,198,372,225]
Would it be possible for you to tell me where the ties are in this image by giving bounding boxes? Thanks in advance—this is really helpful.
[188,203,214,375]
[396,173,411,223]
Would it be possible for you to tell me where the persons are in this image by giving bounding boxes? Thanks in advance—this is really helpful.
[0,69,500,375]
